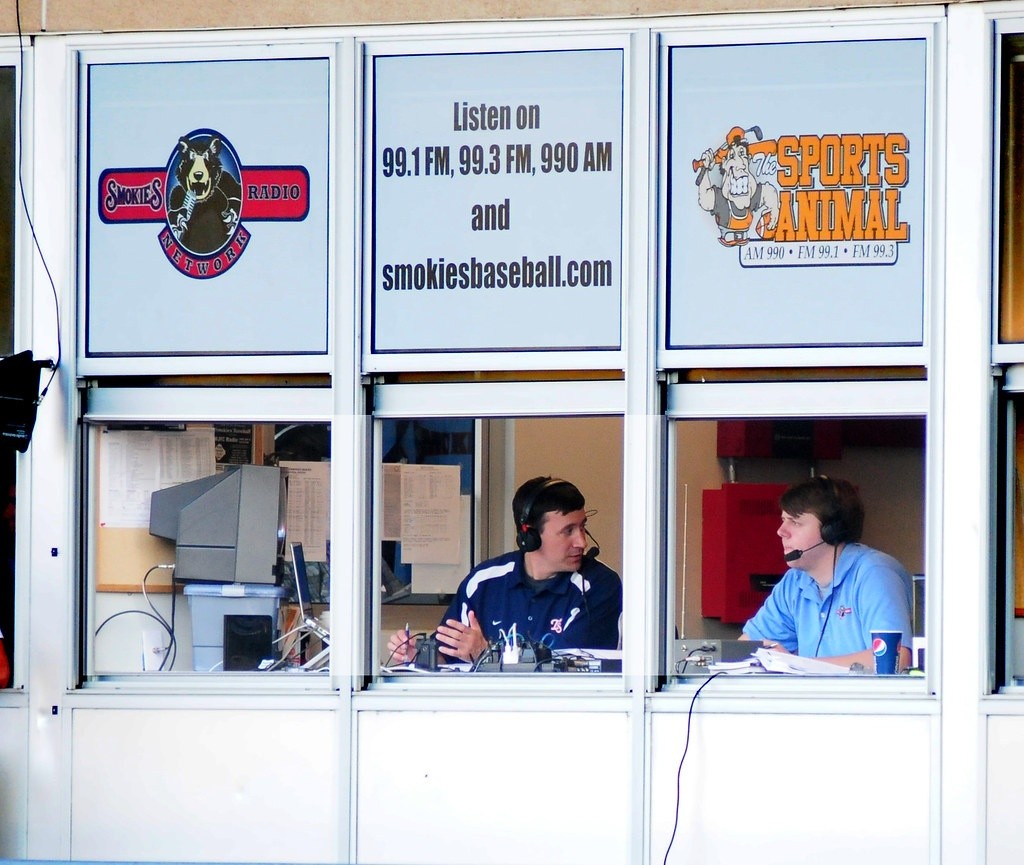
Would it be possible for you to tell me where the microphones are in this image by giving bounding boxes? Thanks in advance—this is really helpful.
[784,531,845,562]
[584,530,600,557]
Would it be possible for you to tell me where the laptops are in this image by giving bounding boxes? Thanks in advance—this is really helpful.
[289,542,330,642]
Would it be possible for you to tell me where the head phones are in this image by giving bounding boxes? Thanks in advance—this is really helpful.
[516,478,565,552]
[820,475,850,547]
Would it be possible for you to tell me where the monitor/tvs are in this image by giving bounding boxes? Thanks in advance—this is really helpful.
[150,463,290,587]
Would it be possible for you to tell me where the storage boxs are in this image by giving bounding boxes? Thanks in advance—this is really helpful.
[183,582,286,671]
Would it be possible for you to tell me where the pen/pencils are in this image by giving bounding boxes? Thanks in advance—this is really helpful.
[762,642,779,651]
[404,620,410,666]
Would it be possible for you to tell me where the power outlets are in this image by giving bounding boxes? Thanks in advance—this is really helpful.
[141,627,165,670]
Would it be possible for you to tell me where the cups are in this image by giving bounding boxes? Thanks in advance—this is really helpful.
[870,630,904,676]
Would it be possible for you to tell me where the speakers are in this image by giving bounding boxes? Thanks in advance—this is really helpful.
[223,614,273,671]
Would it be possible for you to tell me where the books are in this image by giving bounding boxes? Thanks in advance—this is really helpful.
[707,647,849,675]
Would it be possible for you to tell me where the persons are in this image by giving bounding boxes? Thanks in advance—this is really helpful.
[0,629,10,689]
[737,475,913,675]
[386,476,622,673]
